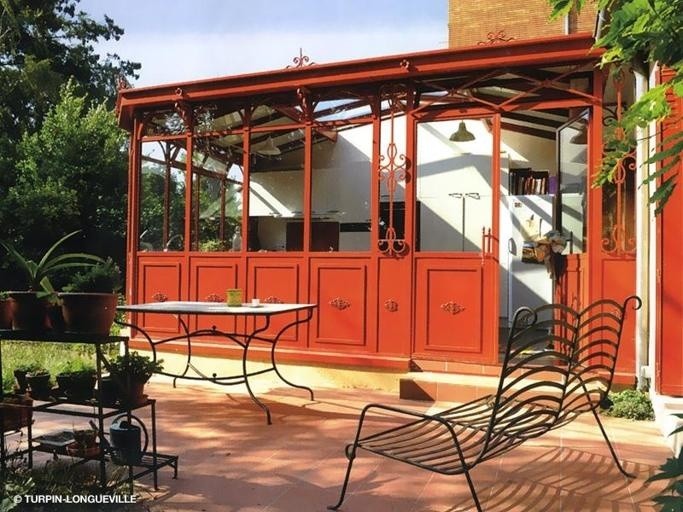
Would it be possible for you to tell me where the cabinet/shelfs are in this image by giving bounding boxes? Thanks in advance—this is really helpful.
[1,325,181,504]
[505,166,588,331]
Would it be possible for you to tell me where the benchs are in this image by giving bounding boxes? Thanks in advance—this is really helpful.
[319,293,644,511]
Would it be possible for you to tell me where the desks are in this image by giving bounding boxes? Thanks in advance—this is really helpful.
[104,300,322,422]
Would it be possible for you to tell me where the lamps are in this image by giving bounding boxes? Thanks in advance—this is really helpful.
[448,119,476,142]
[256,130,281,155]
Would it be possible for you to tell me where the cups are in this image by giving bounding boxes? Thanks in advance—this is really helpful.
[226,288,242,307]
[251,298,260,306]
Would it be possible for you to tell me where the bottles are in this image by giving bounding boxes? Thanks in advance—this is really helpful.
[232,226,242,252]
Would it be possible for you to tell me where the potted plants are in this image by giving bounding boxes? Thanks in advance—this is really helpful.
[0,224,109,333]
[53,254,127,337]
[0,287,16,330]
[1,349,167,465]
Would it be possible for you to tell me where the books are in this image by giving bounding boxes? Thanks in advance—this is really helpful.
[509,171,546,195]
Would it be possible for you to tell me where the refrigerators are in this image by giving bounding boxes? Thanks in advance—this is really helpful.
[508,192,584,331]
